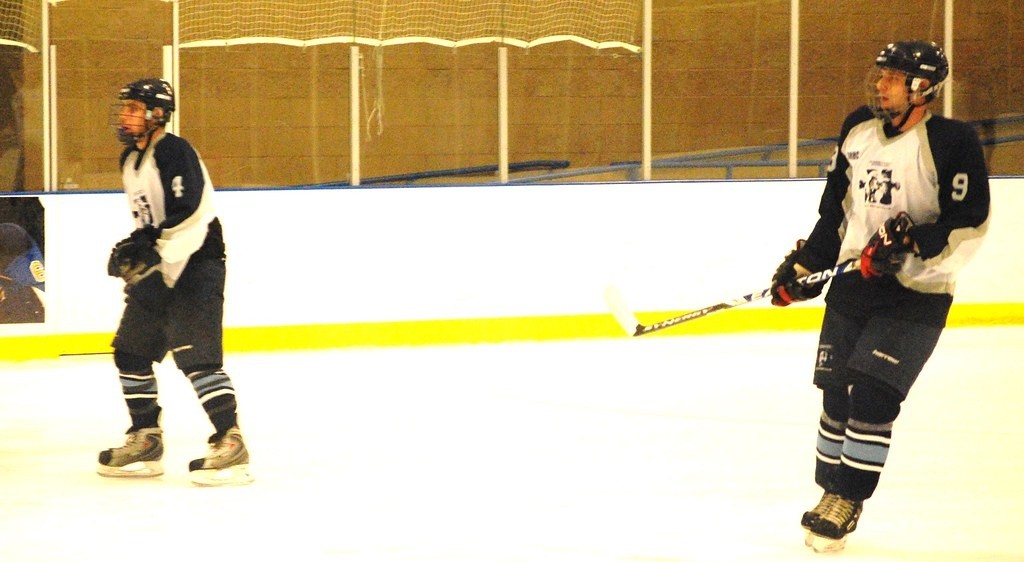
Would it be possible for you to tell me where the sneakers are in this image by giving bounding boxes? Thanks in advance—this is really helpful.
[189,413,255,486]
[96,407,164,477]
[800,490,864,552]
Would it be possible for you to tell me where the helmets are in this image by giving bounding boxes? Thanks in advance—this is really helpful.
[863,39,949,120]
[107,78,175,145]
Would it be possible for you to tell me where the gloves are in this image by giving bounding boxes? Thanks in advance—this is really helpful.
[859,212,915,289]
[108,224,163,286]
[769,239,830,306]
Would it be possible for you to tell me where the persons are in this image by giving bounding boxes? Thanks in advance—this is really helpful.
[98,78,249,472]
[0,223,45,324]
[769,40,993,540]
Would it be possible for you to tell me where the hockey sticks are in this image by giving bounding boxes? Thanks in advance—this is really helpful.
[603,250,908,338]
